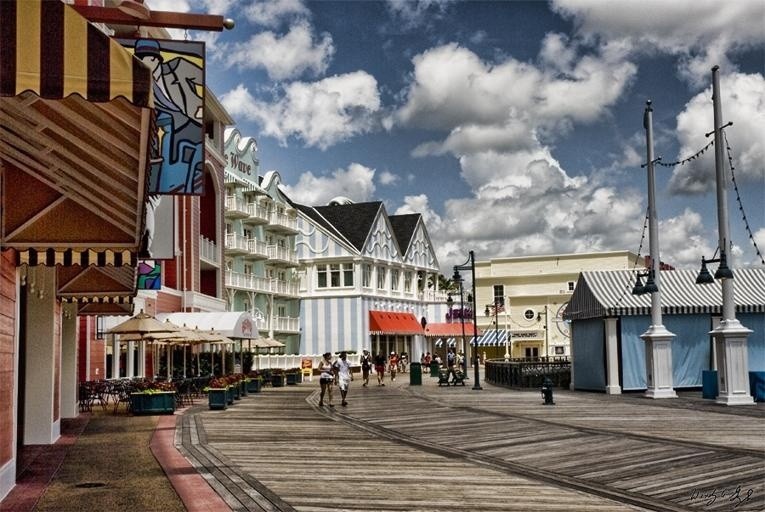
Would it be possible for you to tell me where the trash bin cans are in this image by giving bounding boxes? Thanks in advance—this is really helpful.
[431,361,439,377]
[410,362,421,385]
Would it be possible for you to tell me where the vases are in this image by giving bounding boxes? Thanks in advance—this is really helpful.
[129,394,174,416]
[208,383,242,406]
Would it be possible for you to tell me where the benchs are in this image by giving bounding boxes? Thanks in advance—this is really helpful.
[438,371,464,387]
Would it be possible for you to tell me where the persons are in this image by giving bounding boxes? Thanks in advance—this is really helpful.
[332,351,341,386]
[317,353,336,407]
[482,349,486,365]
[333,351,353,405]
[360,347,466,387]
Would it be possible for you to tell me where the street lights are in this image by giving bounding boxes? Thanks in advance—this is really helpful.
[453,251,483,390]
[447,291,479,379]
[537,306,548,356]
[632,257,663,326]
[485,301,498,358]
[695,235,736,320]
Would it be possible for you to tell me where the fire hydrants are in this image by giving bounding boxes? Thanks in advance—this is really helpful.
[541,378,556,404]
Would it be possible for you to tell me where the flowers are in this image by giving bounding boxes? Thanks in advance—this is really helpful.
[129,368,303,395]
